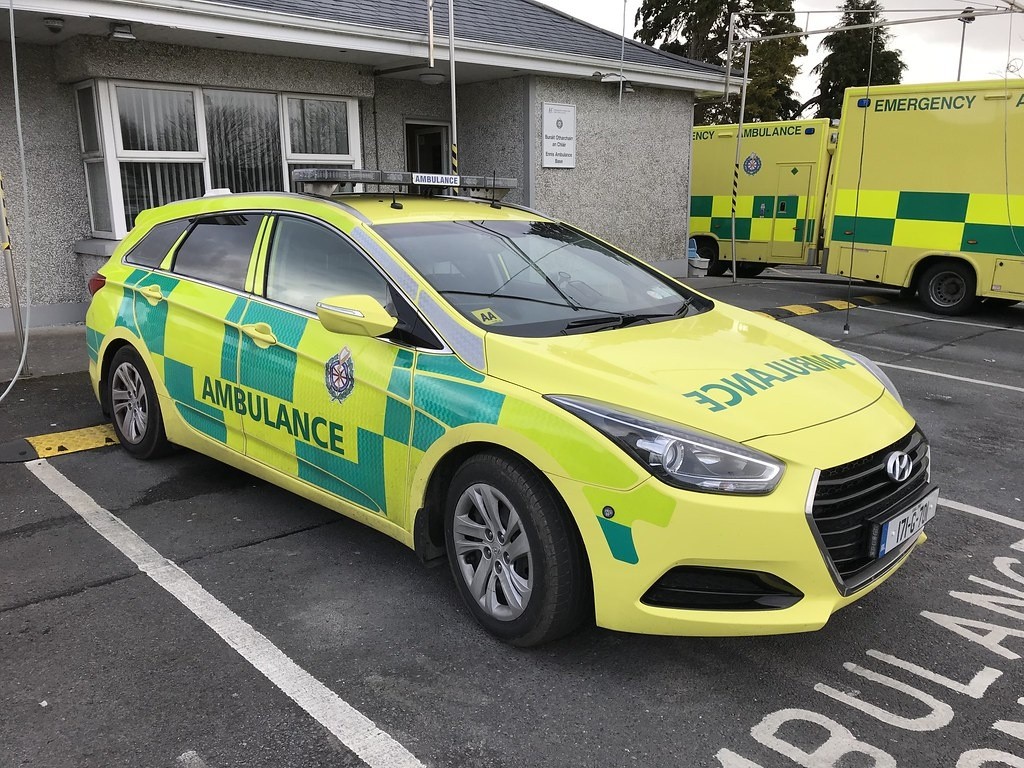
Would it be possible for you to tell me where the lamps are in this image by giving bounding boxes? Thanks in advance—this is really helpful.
[419,73,446,85]
[107,23,136,42]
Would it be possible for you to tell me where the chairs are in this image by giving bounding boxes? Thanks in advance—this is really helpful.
[414,235,479,303]
[312,234,386,300]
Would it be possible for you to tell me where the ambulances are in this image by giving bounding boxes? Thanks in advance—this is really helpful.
[85,169,938,651]
[820,77,1024,314]
[689,118,837,276]
